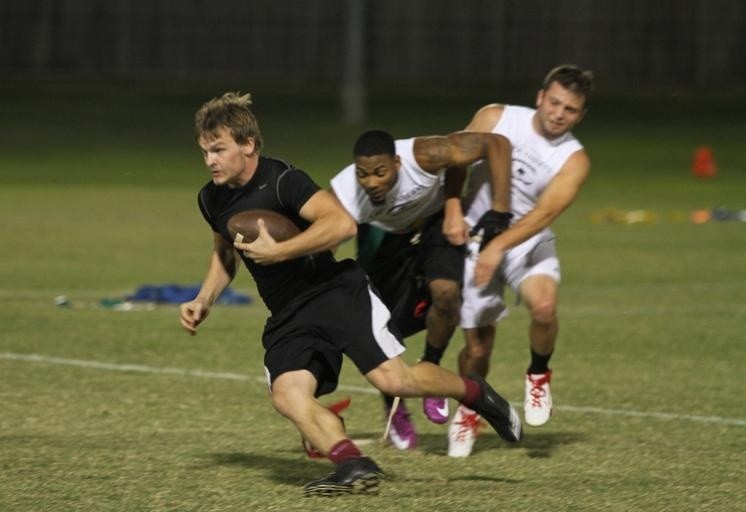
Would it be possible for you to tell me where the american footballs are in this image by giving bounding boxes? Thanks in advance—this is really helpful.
[228,210,302,243]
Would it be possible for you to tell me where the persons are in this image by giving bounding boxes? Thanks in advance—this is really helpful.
[177,90,522,501]
[438,64,593,460]
[324,130,512,453]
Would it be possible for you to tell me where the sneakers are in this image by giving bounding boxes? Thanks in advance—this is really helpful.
[524,368,553,427]
[301,457,385,497]
[446,403,487,459]
[385,403,416,450]
[423,396,450,424]
[459,374,526,445]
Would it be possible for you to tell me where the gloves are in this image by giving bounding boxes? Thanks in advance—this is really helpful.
[469,209,513,252]
